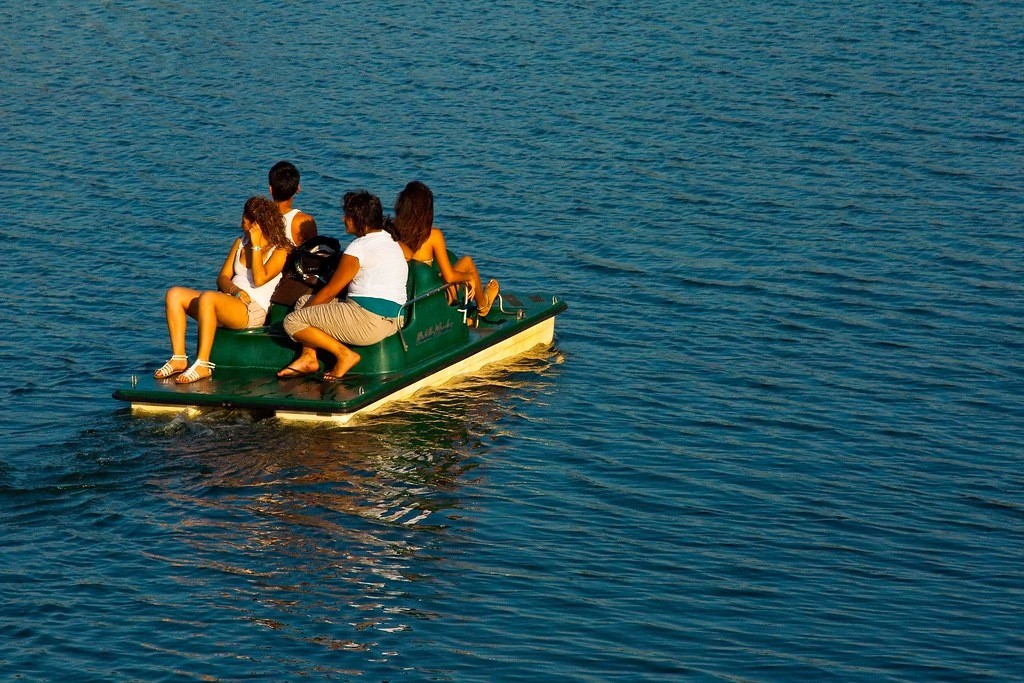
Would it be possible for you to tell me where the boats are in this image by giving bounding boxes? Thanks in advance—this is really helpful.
[112,239,567,431]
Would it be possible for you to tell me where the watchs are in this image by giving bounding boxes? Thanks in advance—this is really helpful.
[251,246,262,251]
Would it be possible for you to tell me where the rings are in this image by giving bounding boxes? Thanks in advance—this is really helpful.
[236,293,240,298]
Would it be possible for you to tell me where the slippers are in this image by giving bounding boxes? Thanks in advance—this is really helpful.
[274,367,322,379]
[319,370,359,382]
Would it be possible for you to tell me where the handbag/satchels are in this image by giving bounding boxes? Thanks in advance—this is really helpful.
[296,235,341,275]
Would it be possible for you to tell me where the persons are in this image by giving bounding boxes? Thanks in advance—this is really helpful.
[268,160,319,248]
[392,180,500,317]
[154,193,296,383]
[278,190,409,381]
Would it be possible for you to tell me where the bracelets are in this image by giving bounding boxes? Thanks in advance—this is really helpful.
[232,286,238,294]
[229,284,235,293]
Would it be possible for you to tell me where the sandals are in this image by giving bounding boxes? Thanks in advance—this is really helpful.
[154,354,189,378]
[174,359,215,383]
[468,288,474,301]
[477,279,500,316]
